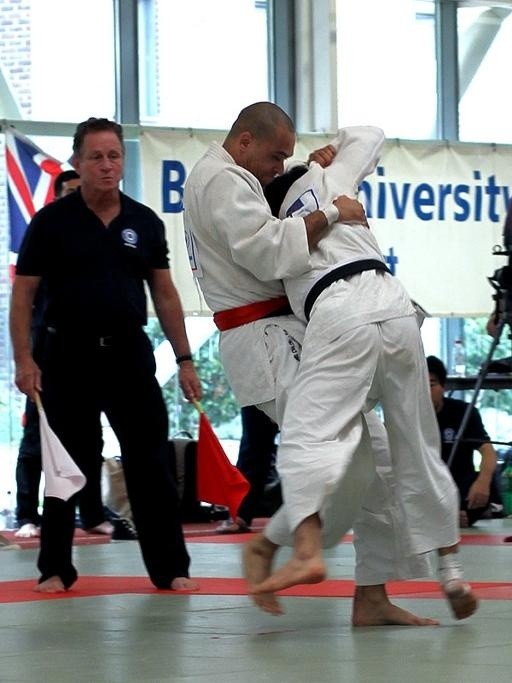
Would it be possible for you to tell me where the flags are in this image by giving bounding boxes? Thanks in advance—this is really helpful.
[5,126,74,287]
[196,412,251,523]
[36,408,88,502]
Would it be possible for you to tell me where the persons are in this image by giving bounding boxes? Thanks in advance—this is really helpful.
[216,404,278,535]
[15,170,115,539]
[184,102,441,627]
[9,117,203,591]
[426,356,497,528]
[247,125,478,618]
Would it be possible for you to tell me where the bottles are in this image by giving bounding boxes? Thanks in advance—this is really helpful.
[451,340,465,375]
[500,463,512,515]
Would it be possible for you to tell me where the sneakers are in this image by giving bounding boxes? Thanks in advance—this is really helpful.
[109,518,138,542]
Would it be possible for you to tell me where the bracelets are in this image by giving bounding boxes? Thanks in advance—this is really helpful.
[176,356,192,364]
[319,204,340,227]
